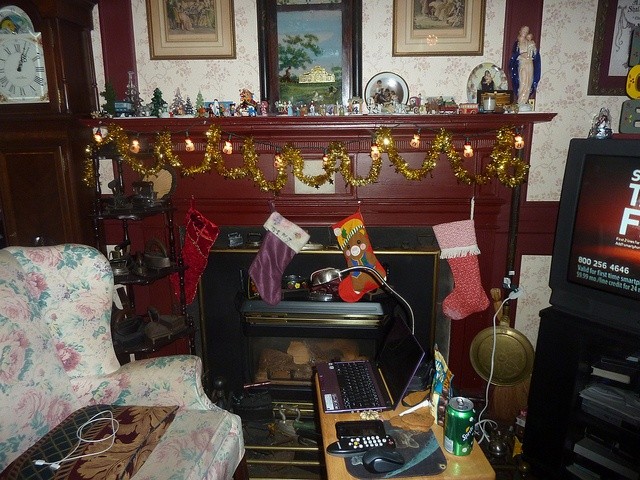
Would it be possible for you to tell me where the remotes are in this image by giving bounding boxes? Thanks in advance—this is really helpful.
[326,434,396,457]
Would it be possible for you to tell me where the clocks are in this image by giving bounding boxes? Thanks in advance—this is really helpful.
[0,5,51,104]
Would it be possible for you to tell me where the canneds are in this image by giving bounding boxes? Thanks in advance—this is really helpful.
[443,396,475,456]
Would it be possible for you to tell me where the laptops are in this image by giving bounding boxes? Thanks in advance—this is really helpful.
[316,314,425,414]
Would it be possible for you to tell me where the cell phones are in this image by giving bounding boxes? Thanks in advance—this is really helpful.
[335,420,392,438]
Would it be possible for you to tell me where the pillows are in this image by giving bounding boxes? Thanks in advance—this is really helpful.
[0,404,180,480]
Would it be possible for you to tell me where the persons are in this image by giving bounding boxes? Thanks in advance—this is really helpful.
[510,24,541,107]
[481,70,494,90]
[374,79,399,105]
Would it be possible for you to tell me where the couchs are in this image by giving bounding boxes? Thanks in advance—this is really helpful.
[0,242,249,480]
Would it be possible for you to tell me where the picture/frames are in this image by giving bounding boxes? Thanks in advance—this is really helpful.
[145,0,237,60]
[392,0,486,57]
[252,0,360,99]
[586,0,640,96]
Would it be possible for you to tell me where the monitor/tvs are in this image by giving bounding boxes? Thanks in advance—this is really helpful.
[546,137,639,338]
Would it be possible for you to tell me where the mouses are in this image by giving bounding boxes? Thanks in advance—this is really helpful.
[363,448,404,472]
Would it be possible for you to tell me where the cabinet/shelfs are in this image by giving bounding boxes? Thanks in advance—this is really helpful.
[95,142,196,362]
[516,307,639,478]
